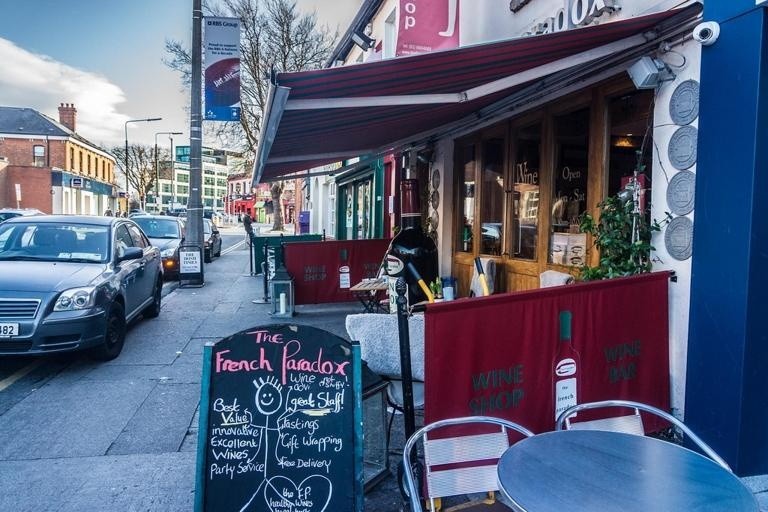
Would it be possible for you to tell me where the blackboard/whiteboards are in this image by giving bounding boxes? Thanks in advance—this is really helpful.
[195,324,364,512]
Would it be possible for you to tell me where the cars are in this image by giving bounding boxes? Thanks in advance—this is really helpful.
[0,206,225,361]
[481,223,501,252]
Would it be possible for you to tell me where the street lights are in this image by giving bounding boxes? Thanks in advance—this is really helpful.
[125,118,163,217]
[155,132,184,212]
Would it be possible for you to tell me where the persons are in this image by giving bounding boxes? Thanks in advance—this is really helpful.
[104,206,112,216]
[242,211,258,243]
[115,210,120,217]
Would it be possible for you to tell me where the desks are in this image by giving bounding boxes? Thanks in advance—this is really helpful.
[350,280,391,315]
[493,427,759,512]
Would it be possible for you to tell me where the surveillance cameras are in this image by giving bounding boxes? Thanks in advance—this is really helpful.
[692,22,721,46]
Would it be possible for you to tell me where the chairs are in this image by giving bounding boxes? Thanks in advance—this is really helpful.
[384,261,587,427]
[553,396,738,478]
[401,411,542,512]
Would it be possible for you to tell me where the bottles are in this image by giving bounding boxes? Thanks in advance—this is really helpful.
[338,248,351,292]
[550,310,582,425]
[385,176,440,315]
